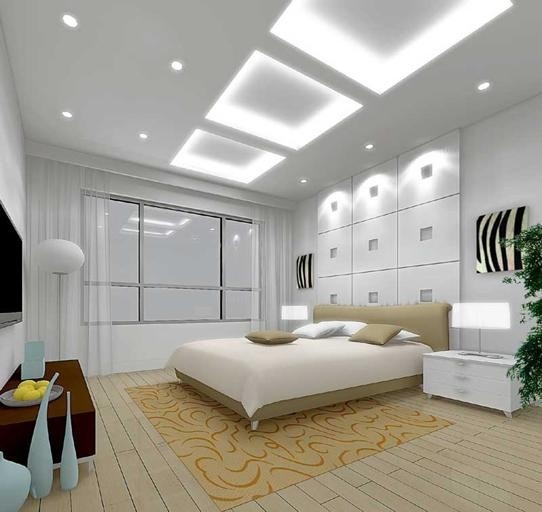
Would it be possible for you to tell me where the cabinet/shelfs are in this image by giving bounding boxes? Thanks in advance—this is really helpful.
[1,358,95,470]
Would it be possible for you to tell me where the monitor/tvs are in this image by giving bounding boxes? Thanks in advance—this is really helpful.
[0,199,22,330]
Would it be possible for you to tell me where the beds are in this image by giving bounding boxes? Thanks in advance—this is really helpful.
[161,302,450,432]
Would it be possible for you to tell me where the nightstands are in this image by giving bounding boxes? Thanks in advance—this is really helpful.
[420,349,524,418]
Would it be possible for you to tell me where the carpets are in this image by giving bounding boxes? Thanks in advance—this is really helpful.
[125,381,456,512]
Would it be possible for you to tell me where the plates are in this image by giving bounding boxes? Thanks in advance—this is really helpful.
[0,385,64,408]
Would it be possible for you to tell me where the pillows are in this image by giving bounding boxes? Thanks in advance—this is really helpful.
[393,330,421,342]
[244,331,298,345]
[292,323,344,338]
[319,320,367,336]
[348,323,403,345]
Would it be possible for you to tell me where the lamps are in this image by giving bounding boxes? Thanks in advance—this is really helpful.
[281,305,307,331]
[452,301,509,360]
[34,237,84,361]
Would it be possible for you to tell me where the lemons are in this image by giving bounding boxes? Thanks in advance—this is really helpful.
[13,379,50,401]
